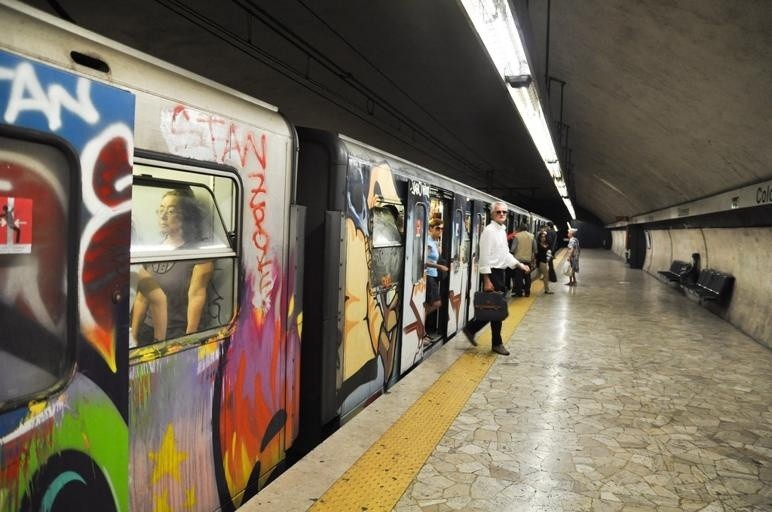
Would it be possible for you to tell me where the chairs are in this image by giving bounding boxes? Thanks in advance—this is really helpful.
[657,259,736,308]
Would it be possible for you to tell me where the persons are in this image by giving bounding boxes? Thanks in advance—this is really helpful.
[132,189,216,345]
[564,228,580,285]
[627,233,633,263]
[127,262,169,349]
[677,254,700,290]
[505,222,557,297]
[461,202,530,356]
[422,218,450,316]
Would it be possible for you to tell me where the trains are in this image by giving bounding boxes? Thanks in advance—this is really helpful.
[0,0,558,512]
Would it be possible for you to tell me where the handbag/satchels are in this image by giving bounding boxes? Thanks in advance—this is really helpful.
[473,290,509,321]
[563,259,573,277]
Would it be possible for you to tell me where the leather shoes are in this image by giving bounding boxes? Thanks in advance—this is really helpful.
[545,290,554,294]
[462,326,477,346]
[510,294,523,298]
[492,343,510,356]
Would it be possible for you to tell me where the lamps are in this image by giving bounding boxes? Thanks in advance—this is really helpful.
[455,0,580,222]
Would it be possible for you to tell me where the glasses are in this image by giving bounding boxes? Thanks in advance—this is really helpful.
[495,211,507,215]
[435,226,445,231]
